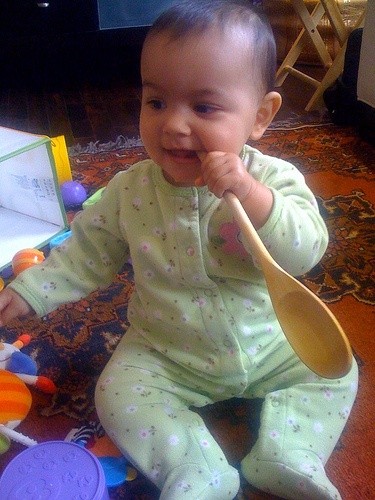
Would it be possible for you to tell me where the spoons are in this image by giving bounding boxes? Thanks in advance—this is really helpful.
[195,150,353,379]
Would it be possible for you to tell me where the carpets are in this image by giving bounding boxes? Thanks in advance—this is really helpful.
[0,112,374,500]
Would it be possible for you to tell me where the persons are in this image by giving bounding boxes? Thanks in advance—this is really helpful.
[0,0,359,499]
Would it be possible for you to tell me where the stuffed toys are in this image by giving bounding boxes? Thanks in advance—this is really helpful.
[0,332,56,453]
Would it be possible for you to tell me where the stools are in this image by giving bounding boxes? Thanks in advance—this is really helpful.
[273,0,366,111]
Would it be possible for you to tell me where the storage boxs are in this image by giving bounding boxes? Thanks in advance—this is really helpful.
[0,441,112,499]
[0,126,68,277]
